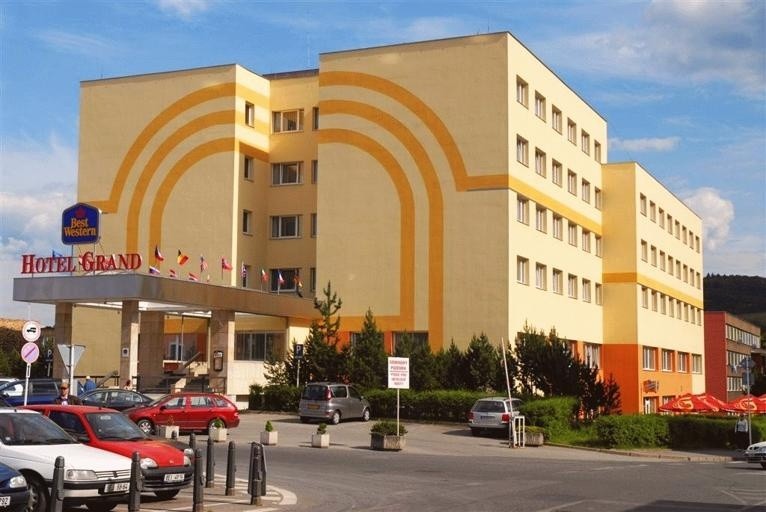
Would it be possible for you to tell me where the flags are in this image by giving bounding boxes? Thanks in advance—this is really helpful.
[146,246,311,297]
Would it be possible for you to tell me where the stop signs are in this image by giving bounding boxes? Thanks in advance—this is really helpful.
[19,342,39,364]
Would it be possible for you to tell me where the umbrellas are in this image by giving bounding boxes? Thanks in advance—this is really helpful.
[662,392,764,420]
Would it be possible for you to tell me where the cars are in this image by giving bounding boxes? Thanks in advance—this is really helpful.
[0,377,23,396]
[469,396,527,436]
[140,427,195,473]
[119,391,239,436]
[13,403,193,500]
[296,382,371,424]
[76,388,154,412]
[0,409,144,511]
[745,441,766,469]
[0,461,29,511]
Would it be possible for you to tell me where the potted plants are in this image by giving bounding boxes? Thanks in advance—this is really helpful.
[368,418,409,450]
[312,422,330,447]
[160,415,180,440]
[260,420,278,445]
[208,418,227,442]
[515,424,548,446]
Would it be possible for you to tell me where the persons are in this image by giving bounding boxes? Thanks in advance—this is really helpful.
[734,413,749,453]
[123,380,135,393]
[54,383,83,407]
[82,376,97,394]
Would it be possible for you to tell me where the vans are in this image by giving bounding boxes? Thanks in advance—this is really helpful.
[0,378,96,408]
